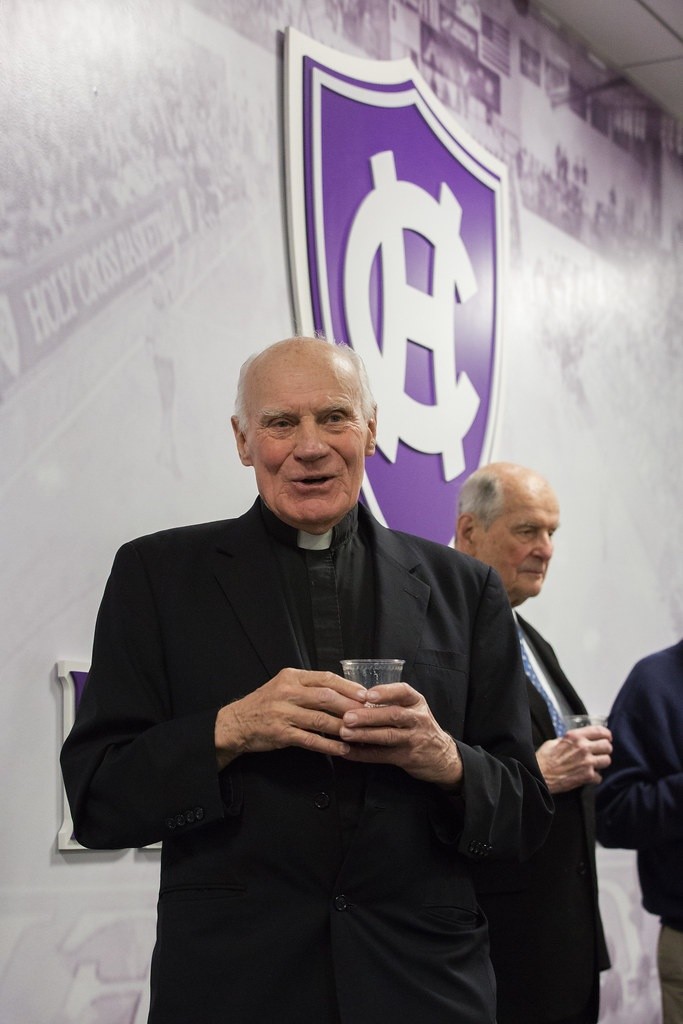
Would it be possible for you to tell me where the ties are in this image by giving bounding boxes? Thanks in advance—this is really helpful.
[518,627,570,739]
[301,546,348,676]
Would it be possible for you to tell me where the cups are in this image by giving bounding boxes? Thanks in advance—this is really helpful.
[341,658,405,711]
[563,714,609,733]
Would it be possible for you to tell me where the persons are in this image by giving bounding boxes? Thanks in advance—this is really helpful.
[455,465,613,1023]
[62,336,554,1022]
[598,638,683,1023]
